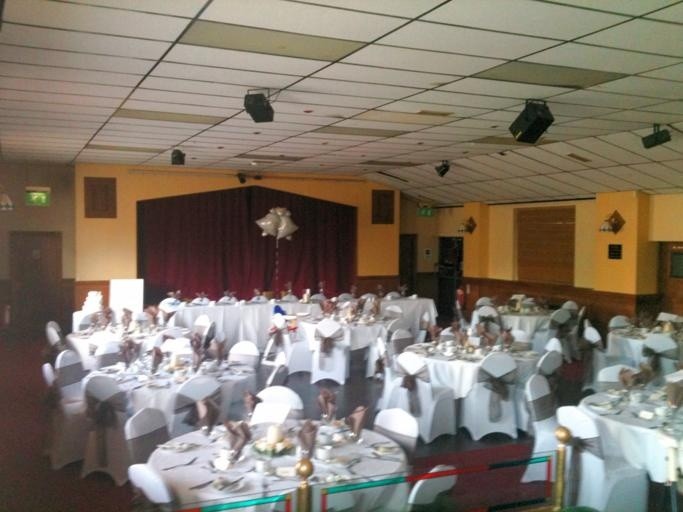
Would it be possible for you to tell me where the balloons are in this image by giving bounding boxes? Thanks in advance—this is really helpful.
[255,206,301,243]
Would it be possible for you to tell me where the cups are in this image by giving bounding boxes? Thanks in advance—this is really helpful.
[447,346,457,353]
[653,406,673,418]
[628,392,643,402]
[446,340,455,347]
[315,445,333,460]
[255,456,272,473]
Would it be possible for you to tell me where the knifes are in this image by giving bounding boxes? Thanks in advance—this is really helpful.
[219,476,244,492]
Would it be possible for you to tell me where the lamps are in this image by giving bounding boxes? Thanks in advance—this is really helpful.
[236,171,247,184]
[601,212,624,234]
[244,87,274,124]
[510,99,556,145]
[435,159,451,176]
[170,148,184,165]
[457,219,476,236]
[643,120,672,148]
[2,156,14,213]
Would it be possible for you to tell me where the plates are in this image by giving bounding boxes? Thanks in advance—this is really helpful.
[213,480,243,493]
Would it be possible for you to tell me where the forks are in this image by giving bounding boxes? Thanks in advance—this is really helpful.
[161,457,198,470]
[599,409,623,417]
